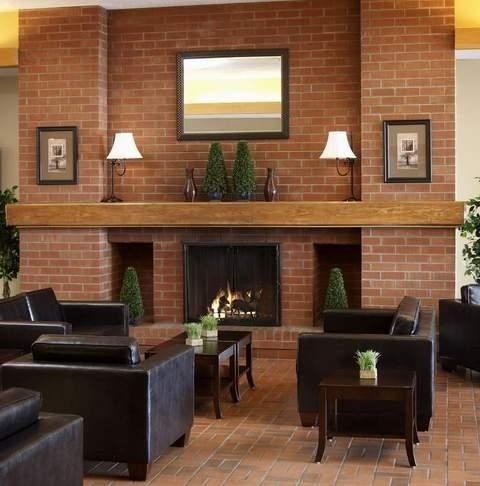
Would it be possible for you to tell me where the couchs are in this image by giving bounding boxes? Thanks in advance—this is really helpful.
[2,387,85,485]
[298,297,435,432]
[1,334,196,482]
[439,283,480,374]
[0,286,129,339]
[0,345,29,363]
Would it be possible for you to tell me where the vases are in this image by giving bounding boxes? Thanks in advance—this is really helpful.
[264,167,277,201]
[184,167,197,202]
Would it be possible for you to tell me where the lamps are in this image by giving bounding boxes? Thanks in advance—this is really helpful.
[106,132,145,201]
[318,130,357,201]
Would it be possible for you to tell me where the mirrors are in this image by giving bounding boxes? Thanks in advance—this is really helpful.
[175,47,289,142]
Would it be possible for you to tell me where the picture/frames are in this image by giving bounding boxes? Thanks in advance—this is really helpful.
[35,125,80,186]
[382,117,433,185]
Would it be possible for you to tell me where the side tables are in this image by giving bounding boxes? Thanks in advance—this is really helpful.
[314,368,422,468]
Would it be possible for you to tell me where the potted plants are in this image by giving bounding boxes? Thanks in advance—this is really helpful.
[231,140,256,201]
[353,348,382,378]
[0,184,26,295]
[120,266,145,324]
[203,142,228,201]
[200,314,220,337]
[458,174,480,285]
[185,322,204,346]
[323,268,349,310]
